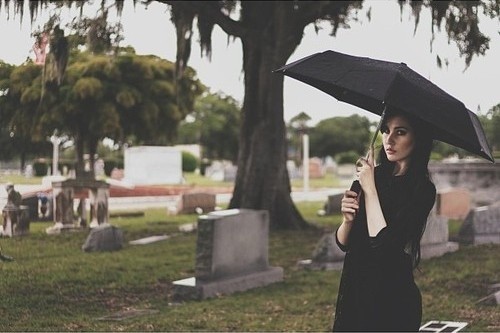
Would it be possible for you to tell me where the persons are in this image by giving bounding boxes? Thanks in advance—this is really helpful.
[6,183,22,207]
[333,110,436,333]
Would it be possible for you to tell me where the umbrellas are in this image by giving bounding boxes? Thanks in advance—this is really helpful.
[271,49,495,216]
